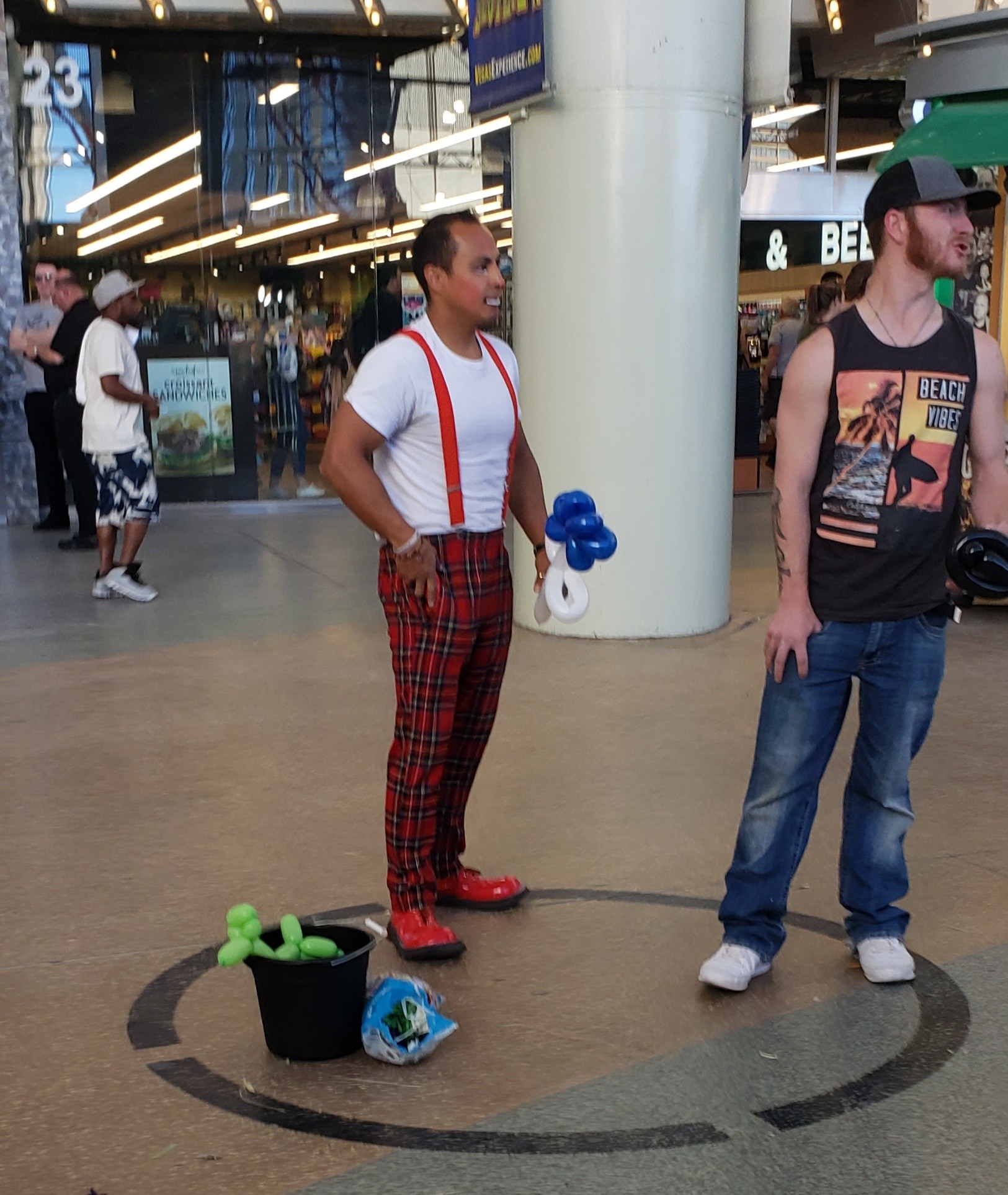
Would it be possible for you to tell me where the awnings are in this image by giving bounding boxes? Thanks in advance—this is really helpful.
[874,101,1008,172]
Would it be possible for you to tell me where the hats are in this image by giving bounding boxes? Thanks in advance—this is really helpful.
[92,269,146,312]
[864,158,1002,226]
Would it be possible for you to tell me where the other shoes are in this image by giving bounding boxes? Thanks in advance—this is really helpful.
[104,562,158,602]
[270,487,291,498]
[32,513,71,531]
[296,485,325,498]
[93,570,126,599]
[58,533,97,551]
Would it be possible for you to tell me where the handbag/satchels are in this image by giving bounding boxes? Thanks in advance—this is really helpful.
[361,971,458,1067]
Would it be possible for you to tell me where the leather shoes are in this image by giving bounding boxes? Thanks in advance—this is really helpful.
[437,868,529,910]
[387,908,466,962]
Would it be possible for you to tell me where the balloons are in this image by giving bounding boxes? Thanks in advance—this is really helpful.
[217,901,345,968]
[532,490,619,625]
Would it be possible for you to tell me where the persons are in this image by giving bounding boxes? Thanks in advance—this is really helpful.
[26,267,100,552]
[317,211,549,962]
[760,296,803,468]
[8,257,72,533]
[795,260,874,343]
[77,271,161,602]
[698,157,1007,992]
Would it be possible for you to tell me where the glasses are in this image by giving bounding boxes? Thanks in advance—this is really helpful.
[35,274,53,281]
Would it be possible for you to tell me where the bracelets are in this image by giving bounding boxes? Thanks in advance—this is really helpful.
[531,543,546,555]
[392,528,420,554]
[33,342,37,358]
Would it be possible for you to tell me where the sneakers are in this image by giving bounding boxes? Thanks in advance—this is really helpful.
[857,936,916,983]
[699,942,771,992]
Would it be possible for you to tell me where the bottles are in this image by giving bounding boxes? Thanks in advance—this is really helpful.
[798,299,807,318]
[739,300,780,314]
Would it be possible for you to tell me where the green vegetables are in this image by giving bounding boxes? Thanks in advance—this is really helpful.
[380,1000,418,1042]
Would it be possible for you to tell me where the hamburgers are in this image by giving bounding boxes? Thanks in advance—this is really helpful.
[154,411,217,467]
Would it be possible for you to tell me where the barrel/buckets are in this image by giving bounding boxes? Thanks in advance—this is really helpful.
[242,918,387,1061]
